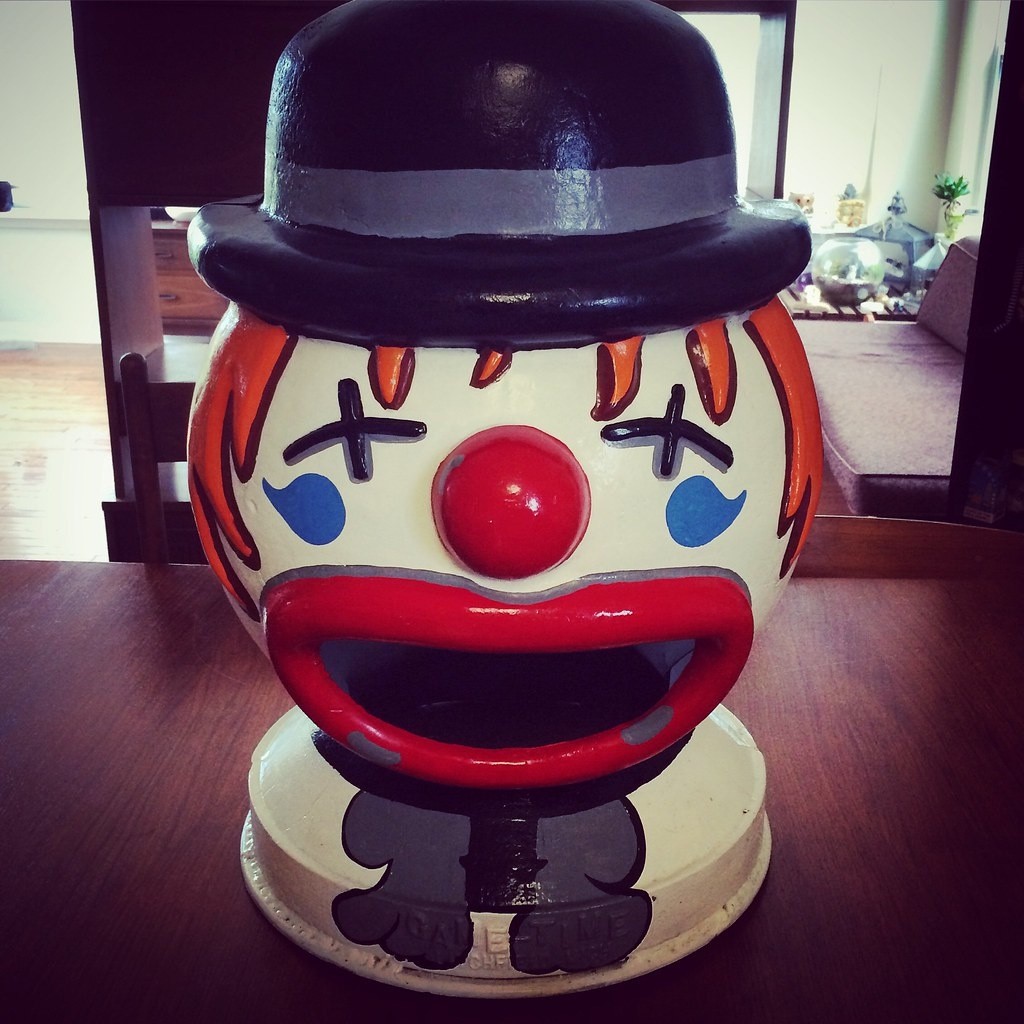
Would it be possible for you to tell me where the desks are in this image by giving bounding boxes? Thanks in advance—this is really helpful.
[0,514,1024,1023]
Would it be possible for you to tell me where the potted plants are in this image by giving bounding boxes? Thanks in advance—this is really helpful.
[931,173,971,239]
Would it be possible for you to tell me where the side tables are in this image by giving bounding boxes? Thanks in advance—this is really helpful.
[779,283,919,321]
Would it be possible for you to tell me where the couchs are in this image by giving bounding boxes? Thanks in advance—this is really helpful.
[794,235,980,519]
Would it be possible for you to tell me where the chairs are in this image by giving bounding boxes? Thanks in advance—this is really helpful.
[119,352,208,565]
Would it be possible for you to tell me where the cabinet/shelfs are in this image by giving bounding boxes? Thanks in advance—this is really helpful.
[152,221,230,326]
